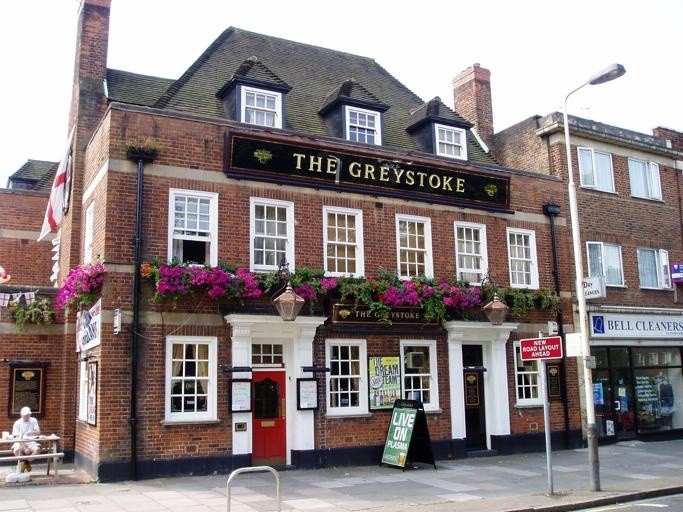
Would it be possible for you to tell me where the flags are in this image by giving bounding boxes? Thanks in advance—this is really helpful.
[24,292,35,306]
[0,293,10,307]
[35,122,76,244]
[12,293,21,306]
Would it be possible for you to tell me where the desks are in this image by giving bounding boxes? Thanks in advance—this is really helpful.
[0,434,60,481]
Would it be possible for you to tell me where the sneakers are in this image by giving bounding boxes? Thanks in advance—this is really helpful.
[20,462,31,473]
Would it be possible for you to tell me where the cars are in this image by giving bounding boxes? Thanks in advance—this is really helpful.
[593,397,671,432]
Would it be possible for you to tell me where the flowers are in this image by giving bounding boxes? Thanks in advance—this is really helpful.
[54,262,106,309]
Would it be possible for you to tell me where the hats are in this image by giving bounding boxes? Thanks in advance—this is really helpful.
[21,407,32,415]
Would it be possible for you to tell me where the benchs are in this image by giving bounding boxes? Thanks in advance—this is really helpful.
[0,446,64,478]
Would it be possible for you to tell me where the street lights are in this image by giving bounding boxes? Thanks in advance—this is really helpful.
[562,61,628,493]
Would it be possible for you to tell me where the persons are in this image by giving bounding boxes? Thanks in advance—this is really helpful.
[88,366,94,392]
[8,405,41,473]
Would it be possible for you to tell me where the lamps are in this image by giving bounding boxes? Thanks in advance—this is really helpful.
[271,259,304,323]
[479,276,510,326]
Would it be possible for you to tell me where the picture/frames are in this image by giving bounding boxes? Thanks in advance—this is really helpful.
[85,360,97,426]
[366,354,402,412]
